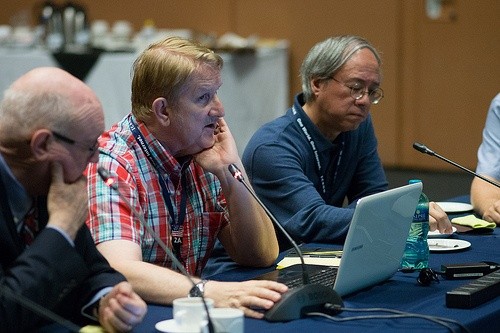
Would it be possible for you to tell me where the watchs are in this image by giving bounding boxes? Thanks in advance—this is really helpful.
[189,278,206,297]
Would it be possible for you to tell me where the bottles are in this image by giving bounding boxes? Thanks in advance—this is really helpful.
[401,179,429,269]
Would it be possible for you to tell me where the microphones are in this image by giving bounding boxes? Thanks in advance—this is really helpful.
[97,165,216,333]
[228,163,344,321]
[412,142,500,189]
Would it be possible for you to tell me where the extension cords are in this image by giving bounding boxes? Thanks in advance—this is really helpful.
[446,269,500,309]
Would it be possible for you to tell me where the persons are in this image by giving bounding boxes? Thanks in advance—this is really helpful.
[468,93,500,223]
[0,66,148,333]
[200,34,451,279]
[83,35,289,322]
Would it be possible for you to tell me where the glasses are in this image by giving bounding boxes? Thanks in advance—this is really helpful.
[52,131,99,153]
[331,76,385,105]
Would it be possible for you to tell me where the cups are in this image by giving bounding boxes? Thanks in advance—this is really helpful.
[155,319,209,333]
[201,308,245,333]
[172,298,214,319]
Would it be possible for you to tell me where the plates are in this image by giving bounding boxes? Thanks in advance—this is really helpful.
[428,226,457,237]
[427,239,471,251]
[419,202,473,212]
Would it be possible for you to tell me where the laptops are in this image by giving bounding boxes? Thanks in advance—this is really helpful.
[243,182,423,299]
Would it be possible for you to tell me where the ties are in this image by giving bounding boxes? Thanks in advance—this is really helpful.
[22,197,38,250]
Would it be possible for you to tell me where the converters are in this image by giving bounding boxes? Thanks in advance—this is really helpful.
[440,261,490,280]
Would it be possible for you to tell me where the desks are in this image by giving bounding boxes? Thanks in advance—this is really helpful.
[0,37,290,161]
[35,195,500,333]
